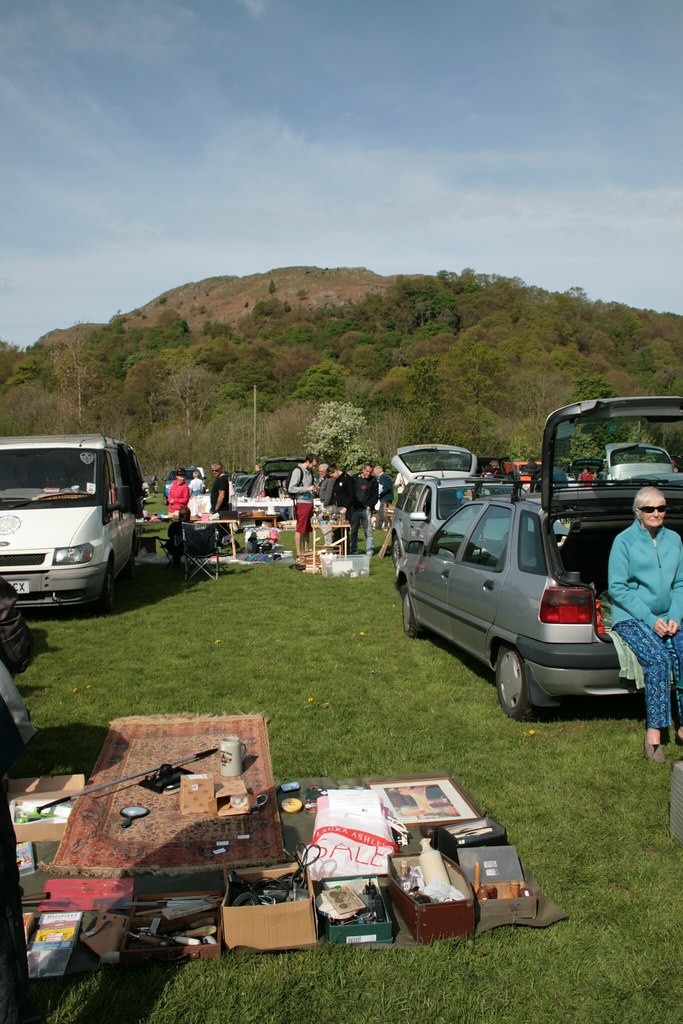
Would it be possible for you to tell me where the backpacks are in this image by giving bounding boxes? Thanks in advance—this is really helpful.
[286,466,314,499]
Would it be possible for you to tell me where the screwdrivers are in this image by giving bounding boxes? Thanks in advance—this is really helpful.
[129,916,217,945]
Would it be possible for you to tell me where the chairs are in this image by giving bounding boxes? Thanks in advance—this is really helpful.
[171,522,220,582]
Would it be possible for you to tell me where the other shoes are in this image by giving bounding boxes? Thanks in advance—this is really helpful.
[366,551,373,557]
[296,554,301,564]
[351,548,359,554]
[645,735,666,764]
[675,733,683,746]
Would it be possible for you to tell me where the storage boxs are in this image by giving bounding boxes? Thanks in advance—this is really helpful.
[179,773,216,815]
[208,780,254,817]
[119,891,222,962]
[218,861,317,953]
[437,816,507,863]
[386,852,477,944]
[7,773,86,842]
[474,884,537,920]
[320,554,370,578]
[321,876,394,946]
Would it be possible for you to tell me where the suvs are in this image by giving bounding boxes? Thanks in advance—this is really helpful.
[391,443,568,579]
[398,395,683,722]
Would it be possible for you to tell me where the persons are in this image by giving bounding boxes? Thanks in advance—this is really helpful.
[581,466,597,486]
[480,460,520,481]
[288,454,379,562]
[165,507,196,567]
[279,481,291,520]
[526,461,568,493]
[188,472,206,496]
[255,464,261,474]
[372,467,394,531]
[608,487,683,764]
[385,785,450,807]
[395,463,410,499]
[210,462,242,554]
[168,468,191,516]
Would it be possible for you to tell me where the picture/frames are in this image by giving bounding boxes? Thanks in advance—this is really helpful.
[365,776,482,826]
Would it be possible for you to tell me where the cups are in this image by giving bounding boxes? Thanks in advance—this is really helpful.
[220,736,247,776]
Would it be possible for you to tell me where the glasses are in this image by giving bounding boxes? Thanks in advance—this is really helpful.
[212,468,220,472]
[637,505,668,513]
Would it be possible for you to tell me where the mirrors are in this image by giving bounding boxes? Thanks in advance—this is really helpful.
[120,805,150,828]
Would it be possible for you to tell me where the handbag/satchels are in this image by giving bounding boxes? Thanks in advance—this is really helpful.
[301,808,399,881]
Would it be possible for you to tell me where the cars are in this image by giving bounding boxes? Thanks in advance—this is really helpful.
[477,442,683,497]
[162,467,207,506]
[231,455,316,527]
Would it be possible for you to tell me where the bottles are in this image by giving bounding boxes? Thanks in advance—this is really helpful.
[510,882,520,897]
[398,860,413,894]
[418,838,450,886]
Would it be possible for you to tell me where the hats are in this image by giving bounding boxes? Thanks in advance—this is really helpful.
[176,468,186,475]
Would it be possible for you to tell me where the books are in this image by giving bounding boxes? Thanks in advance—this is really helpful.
[27,911,83,977]
[16,842,35,877]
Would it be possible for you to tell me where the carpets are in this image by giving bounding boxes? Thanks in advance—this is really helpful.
[52,711,288,877]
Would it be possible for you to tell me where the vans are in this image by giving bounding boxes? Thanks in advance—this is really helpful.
[1,433,148,615]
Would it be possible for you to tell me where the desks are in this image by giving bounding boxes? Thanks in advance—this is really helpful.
[311,522,351,574]
[195,521,237,557]
[240,513,278,529]
